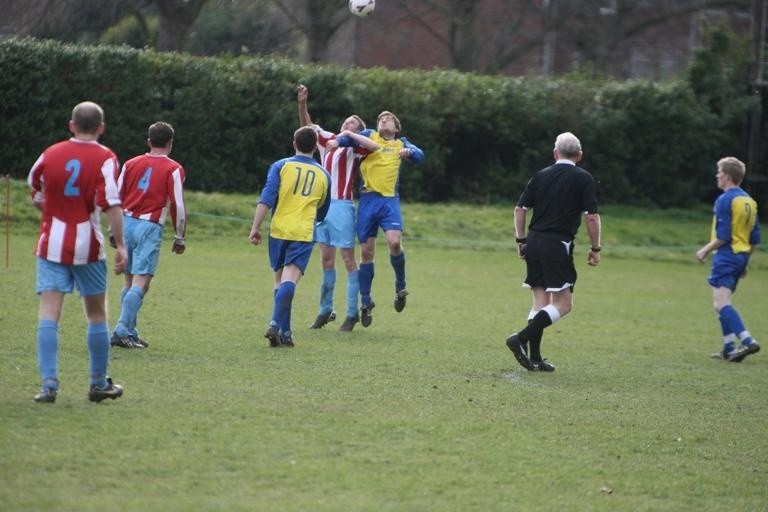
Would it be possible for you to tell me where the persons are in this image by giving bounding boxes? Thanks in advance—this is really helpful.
[249,126,332,348]
[696,157,760,362]
[110,122,187,350]
[506,132,601,372]
[27,101,128,404]
[325,111,425,327]
[297,84,380,332]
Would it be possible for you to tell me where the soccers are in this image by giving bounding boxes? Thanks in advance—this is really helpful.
[348,0,376,17]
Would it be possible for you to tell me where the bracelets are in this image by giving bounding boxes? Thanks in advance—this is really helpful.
[591,245,601,252]
[516,237,527,243]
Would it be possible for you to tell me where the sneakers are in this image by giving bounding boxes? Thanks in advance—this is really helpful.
[340,313,360,332]
[528,359,555,373]
[34,389,56,404]
[361,302,375,327]
[709,340,759,363]
[265,325,294,347]
[111,331,148,349]
[309,310,336,330]
[89,378,123,402]
[394,287,409,313]
[506,333,534,370]
